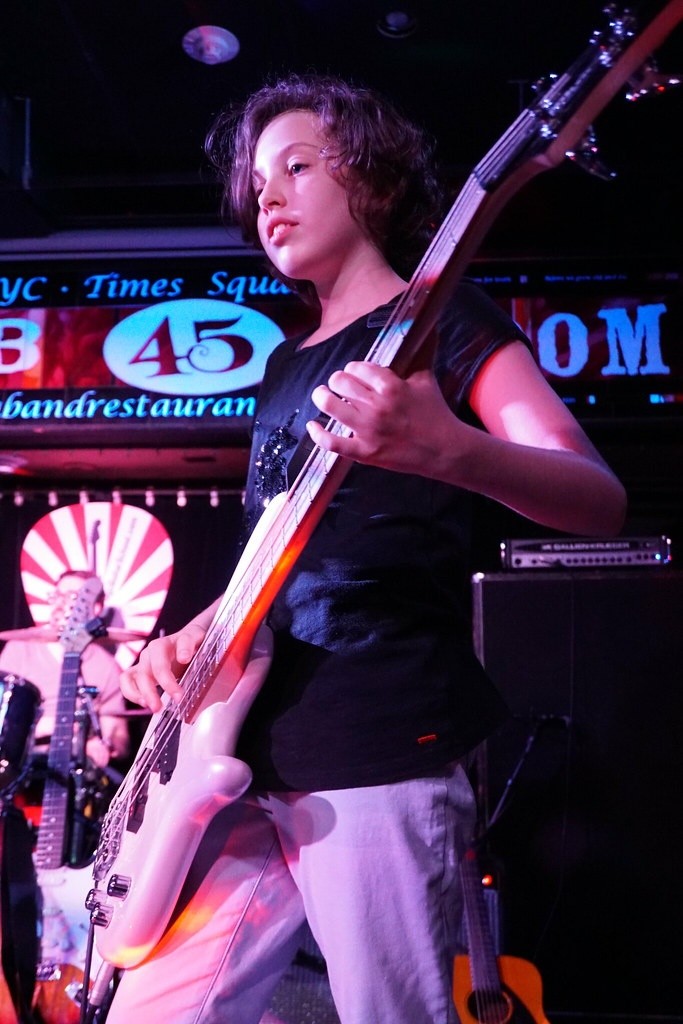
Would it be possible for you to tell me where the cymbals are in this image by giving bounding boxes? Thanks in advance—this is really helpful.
[1,622,150,647]
[103,707,152,718]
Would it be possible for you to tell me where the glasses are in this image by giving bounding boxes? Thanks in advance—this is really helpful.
[48,591,78,604]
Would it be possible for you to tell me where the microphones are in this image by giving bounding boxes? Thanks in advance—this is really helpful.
[532,714,574,735]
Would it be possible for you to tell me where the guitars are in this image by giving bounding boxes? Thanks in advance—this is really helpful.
[89,1,683,968]
[24,577,105,1024]
[454,838,548,1024]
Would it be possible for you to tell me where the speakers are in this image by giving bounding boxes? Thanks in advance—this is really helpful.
[472,572,683,1024]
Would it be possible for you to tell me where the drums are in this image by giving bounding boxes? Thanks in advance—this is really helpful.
[0,667,44,787]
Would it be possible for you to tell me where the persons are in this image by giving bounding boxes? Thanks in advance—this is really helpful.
[0,571,130,805]
[101,59,629,1024]
